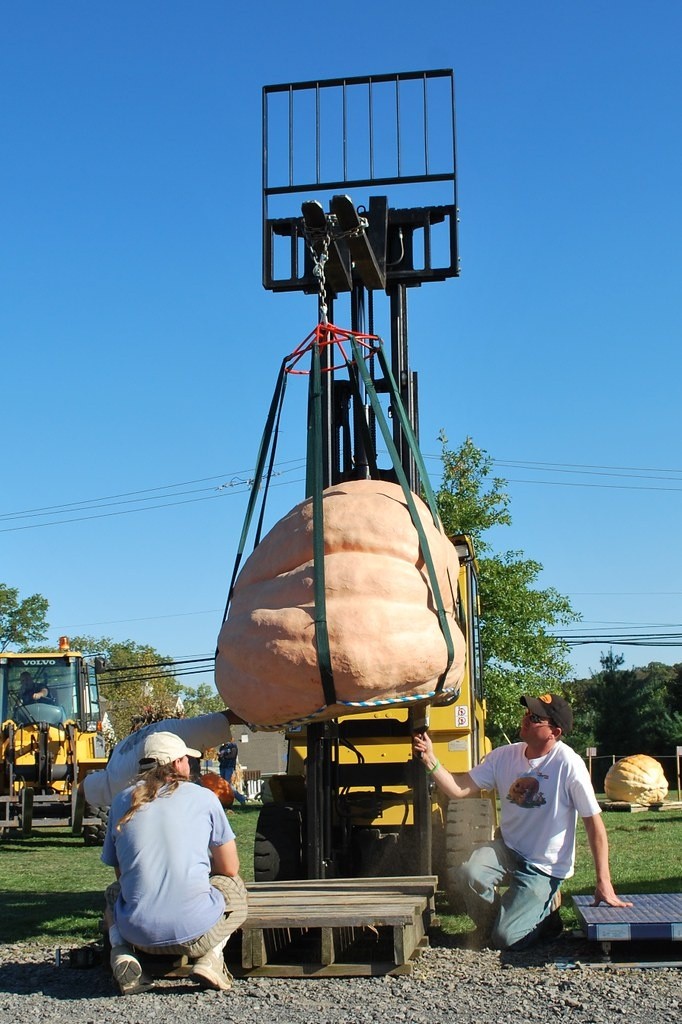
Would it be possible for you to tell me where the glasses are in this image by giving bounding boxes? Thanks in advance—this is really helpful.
[523,707,556,729]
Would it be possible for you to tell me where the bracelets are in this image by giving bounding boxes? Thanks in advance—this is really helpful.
[425,759,440,776]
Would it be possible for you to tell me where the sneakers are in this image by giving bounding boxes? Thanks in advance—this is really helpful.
[189,946,232,991]
[110,944,156,994]
[544,889,563,938]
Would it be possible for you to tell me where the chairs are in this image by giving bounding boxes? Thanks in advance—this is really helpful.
[49,687,58,706]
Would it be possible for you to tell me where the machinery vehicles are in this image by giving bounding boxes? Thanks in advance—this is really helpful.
[254,66,499,917]
[0,636,110,847]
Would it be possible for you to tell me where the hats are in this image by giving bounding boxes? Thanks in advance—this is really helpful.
[520,693,574,736]
[138,731,202,769]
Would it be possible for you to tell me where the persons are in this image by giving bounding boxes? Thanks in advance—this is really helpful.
[216,737,247,805]
[15,672,51,708]
[412,693,634,952]
[100,731,248,997]
